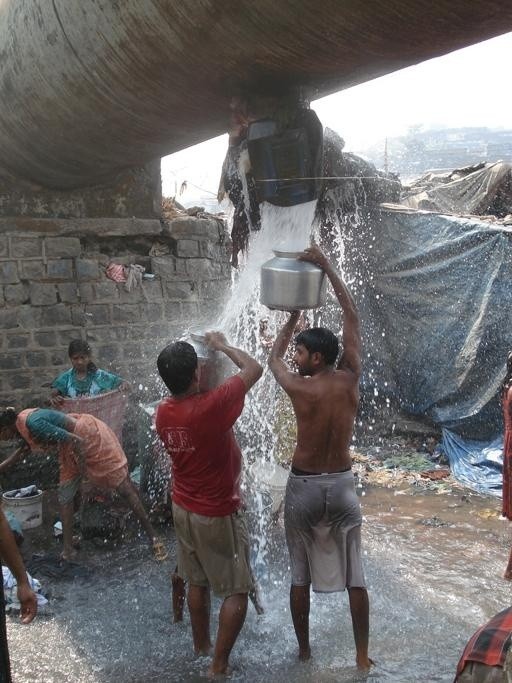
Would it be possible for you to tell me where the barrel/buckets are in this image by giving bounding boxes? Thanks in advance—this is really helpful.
[244,489,274,534]
[261,249,327,310]
[246,108,323,207]
[3,490,42,529]
[249,461,289,512]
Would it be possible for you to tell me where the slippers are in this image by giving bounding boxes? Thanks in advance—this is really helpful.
[152,543,168,561]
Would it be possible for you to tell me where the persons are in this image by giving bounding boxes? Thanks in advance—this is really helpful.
[0,340,169,561]
[502,351,512,581]
[454,607,512,683]
[0,508,37,683]
[268,233,376,670]
[157,331,263,681]
